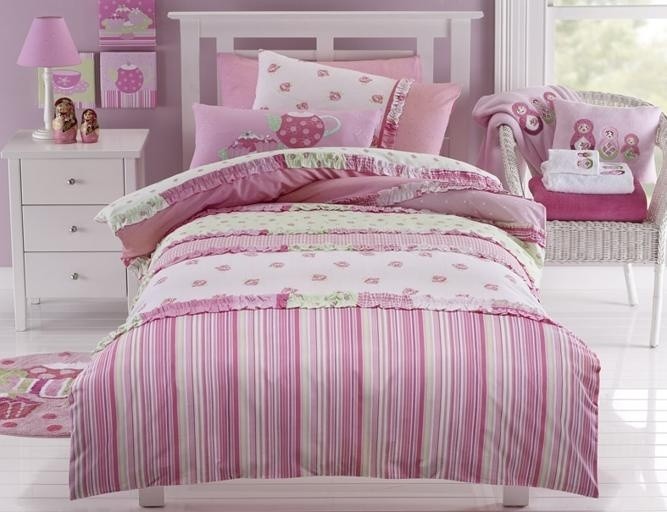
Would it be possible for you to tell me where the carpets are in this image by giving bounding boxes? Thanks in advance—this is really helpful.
[0,352,92,436]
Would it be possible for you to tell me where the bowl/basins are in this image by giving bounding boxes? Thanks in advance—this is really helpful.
[53,69,81,90]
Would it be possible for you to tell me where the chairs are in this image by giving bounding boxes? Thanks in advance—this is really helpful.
[498,91,667,348]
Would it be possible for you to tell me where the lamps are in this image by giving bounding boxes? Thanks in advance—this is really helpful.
[16,15,83,140]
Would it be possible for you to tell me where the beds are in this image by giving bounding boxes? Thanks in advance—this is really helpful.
[69,12,601,507]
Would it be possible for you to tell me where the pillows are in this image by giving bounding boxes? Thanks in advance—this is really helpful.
[551,98,662,180]
[217,52,420,110]
[188,102,380,169]
[253,48,462,155]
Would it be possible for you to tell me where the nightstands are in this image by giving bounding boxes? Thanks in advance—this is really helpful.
[0,128,150,333]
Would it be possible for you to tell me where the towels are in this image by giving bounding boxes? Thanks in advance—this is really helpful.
[539,161,634,195]
[548,147,599,175]
